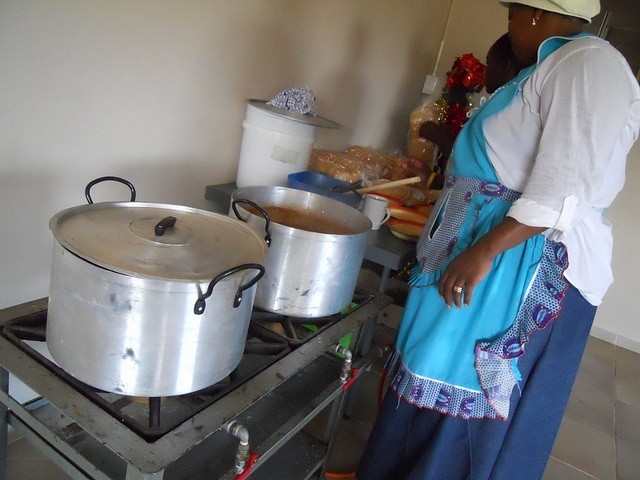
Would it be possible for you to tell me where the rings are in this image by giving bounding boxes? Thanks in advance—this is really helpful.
[454,286,462,293]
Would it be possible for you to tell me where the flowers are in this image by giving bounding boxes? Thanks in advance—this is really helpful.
[436,49,488,143]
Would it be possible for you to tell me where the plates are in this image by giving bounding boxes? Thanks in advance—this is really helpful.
[386,205,429,226]
[387,219,429,242]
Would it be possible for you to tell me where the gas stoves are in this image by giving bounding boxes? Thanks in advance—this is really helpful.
[1,287,392,480]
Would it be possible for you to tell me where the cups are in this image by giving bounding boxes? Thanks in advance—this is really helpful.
[360,193,391,231]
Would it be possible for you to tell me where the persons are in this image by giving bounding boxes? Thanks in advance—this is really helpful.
[418,32,524,185]
[356,0,640,480]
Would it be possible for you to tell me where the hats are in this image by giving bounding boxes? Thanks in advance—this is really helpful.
[499,0,601,23]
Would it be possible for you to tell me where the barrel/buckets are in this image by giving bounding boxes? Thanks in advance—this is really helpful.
[235,105,317,189]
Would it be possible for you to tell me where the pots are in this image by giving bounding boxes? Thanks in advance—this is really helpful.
[228,184,373,319]
[44,177,268,399]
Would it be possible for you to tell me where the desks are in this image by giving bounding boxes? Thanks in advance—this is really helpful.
[204,178,416,421]
